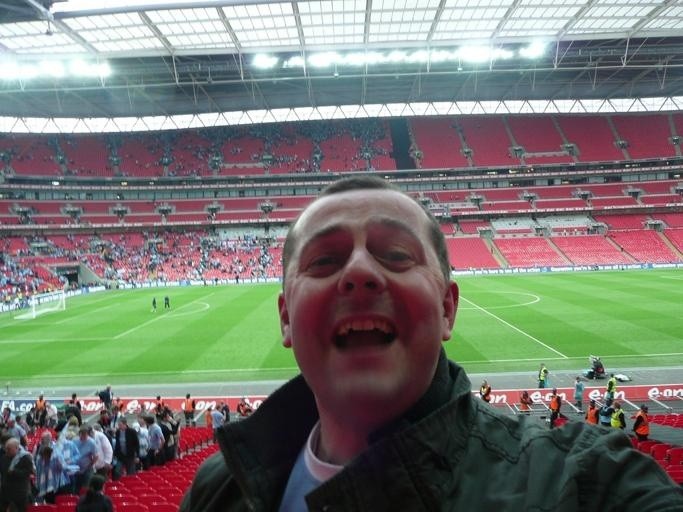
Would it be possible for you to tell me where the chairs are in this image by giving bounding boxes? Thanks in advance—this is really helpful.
[0,424,225,512]
[629,413,683,484]
[0,112,683,302]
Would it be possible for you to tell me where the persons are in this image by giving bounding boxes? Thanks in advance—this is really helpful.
[538,363,549,388]
[548,388,561,429]
[0,383,253,512]
[180,174,683,512]
[479,379,491,402]
[632,404,650,441]
[0,117,388,312]
[574,374,626,430]
[519,391,533,410]
